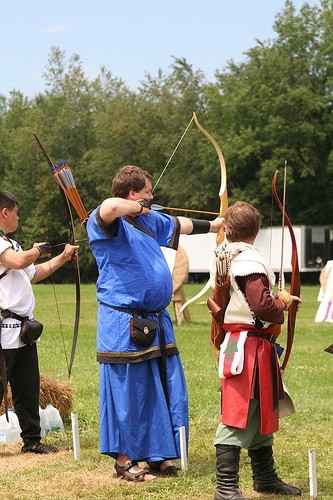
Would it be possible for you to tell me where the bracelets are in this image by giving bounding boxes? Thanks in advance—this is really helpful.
[276,289,293,310]
[33,246,43,256]
[137,201,144,213]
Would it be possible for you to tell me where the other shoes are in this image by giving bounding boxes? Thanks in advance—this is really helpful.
[21,442,60,455]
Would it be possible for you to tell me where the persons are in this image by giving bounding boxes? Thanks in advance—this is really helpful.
[0,190,80,455]
[86,165,226,483]
[214,200,303,500]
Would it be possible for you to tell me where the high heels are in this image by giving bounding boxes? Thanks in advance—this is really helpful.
[146,460,184,475]
[114,461,157,482]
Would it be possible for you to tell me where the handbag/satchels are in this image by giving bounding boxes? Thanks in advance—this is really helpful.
[129,317,158,347]
[19,320,43,345]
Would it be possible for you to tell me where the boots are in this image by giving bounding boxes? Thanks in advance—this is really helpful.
[214,444,246,500]
[248,446,302,496]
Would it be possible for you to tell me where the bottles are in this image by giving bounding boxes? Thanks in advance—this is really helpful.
[0,402,64,444]
